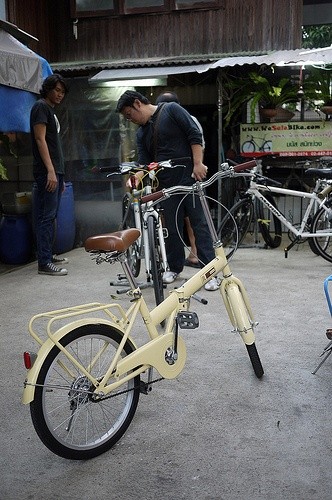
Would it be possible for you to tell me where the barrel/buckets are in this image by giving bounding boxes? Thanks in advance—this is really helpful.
[31,181,75,255]
[0,212,31,266]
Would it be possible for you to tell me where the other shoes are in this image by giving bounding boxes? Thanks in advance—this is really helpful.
[205,276,220,291]
[163,271,177,283]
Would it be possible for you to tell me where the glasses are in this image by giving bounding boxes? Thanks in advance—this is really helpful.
[124,103,134,120]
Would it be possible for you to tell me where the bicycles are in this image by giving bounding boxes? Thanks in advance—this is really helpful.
[105,157,190,308]
[214,157,332,265]
[22,156,265,463]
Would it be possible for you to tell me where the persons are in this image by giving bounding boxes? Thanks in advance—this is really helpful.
[155,91,205,268]
[116,90,219,291]
[30,74,69,275]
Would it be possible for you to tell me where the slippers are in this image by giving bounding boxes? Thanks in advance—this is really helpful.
[185,256,204,267]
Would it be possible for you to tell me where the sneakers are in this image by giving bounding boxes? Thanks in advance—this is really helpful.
[38,262,68,275]
[52,257,69,265]
